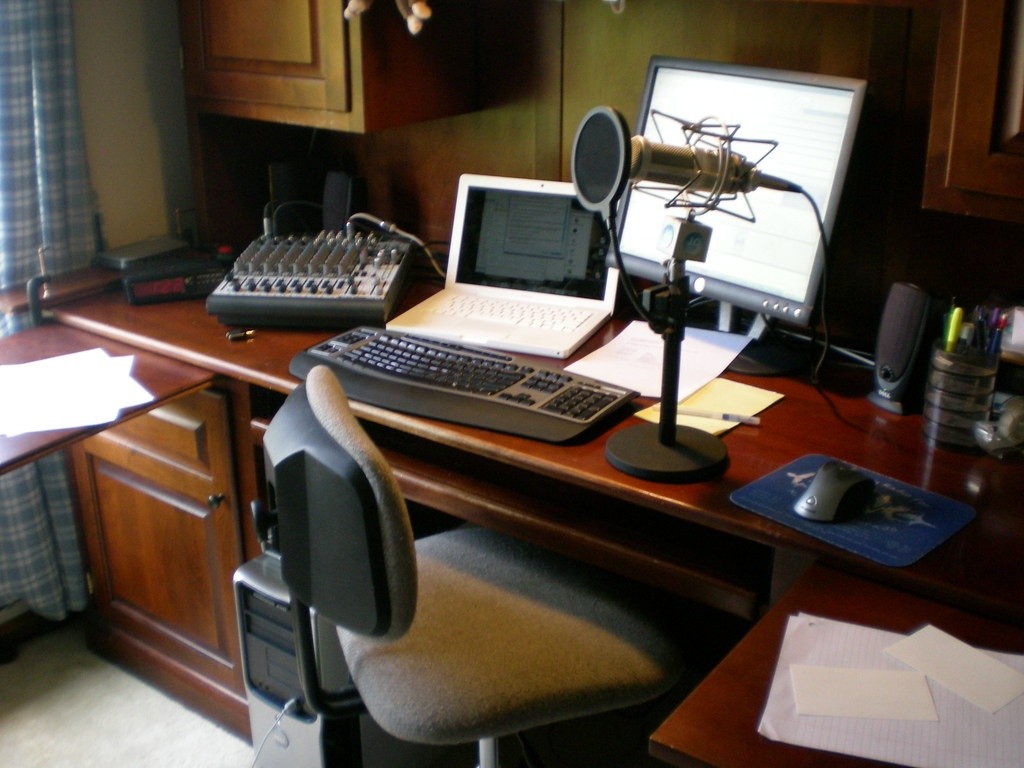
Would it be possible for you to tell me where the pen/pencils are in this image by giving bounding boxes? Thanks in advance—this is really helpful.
[941,298,1009,355]
[655,405,760,425]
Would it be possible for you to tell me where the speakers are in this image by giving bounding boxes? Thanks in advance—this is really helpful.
[867,280,946,414]
[320,176,368,234]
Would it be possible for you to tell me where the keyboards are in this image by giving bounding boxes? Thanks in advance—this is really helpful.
[290,325,640,444]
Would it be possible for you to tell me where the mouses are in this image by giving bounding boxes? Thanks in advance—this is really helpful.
[792,459,878,522]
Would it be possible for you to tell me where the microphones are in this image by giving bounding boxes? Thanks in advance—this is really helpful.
[625,135,802,194]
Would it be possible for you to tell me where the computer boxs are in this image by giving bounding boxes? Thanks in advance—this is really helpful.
[233,552,552,768]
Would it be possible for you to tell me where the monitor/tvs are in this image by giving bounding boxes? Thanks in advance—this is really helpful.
[605,54,868,374]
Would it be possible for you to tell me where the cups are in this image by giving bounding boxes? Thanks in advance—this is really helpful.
[920,337,1002,455]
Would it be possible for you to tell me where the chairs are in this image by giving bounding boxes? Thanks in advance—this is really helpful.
[253,364,687,767]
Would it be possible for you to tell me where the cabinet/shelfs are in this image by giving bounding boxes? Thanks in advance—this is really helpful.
[173,0,489,137]
[910,0,1023,230]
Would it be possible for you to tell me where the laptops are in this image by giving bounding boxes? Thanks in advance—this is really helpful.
[384,174,620,358]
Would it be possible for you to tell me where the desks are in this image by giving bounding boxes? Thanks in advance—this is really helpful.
[52,233,1023,768]
